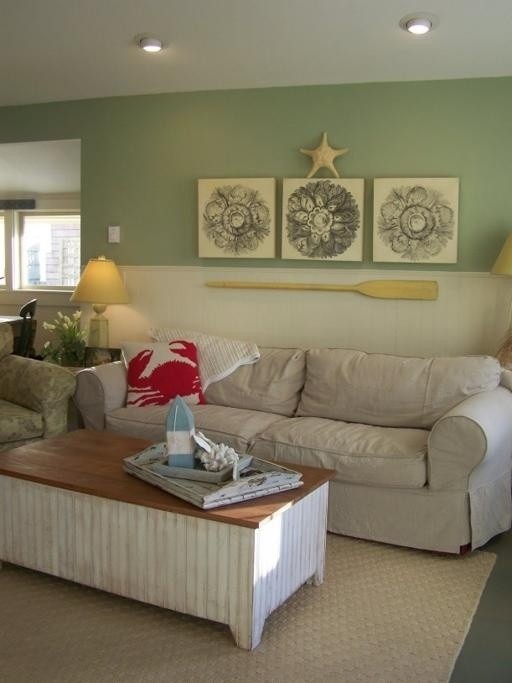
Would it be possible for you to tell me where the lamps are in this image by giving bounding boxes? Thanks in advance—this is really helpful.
[399,11,439,35]
[69,254,129,364]
[132,31,172,54]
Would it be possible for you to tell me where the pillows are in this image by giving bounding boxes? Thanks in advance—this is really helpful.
[118,340,205,408]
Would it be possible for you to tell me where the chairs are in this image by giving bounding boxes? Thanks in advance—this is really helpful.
[0,297,76,456]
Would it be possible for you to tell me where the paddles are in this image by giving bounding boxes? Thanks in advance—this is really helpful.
[206,280,438,301]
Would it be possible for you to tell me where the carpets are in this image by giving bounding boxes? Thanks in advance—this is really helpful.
[0,532,499,683]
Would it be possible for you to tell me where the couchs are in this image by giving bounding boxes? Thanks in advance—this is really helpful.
[73,345,512,557]
